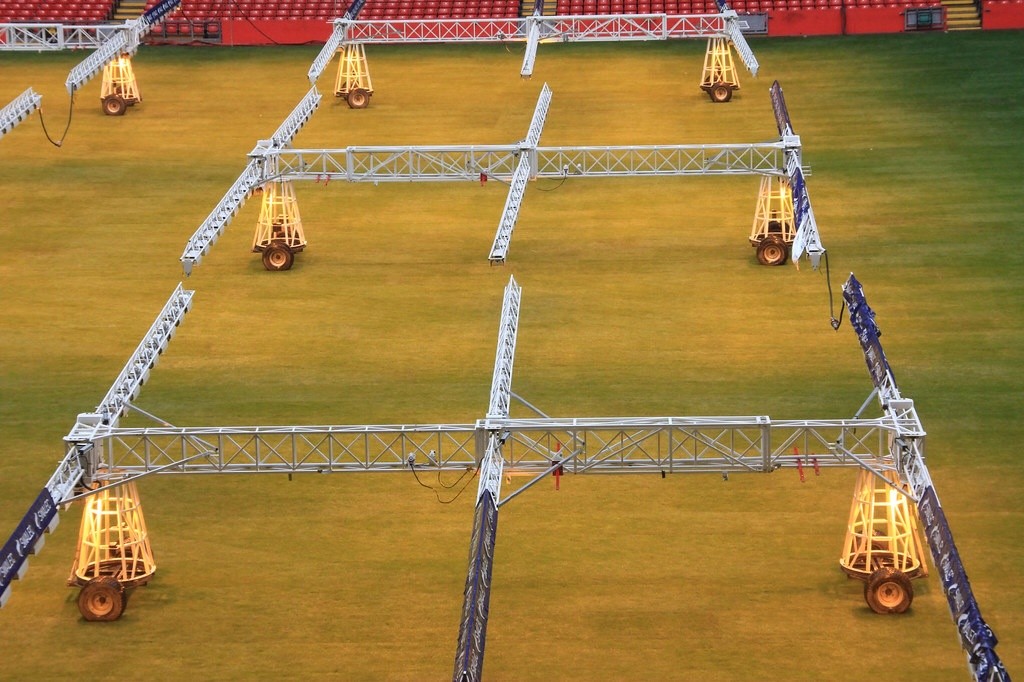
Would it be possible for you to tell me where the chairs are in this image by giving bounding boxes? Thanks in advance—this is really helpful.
[0,0,944,36]
